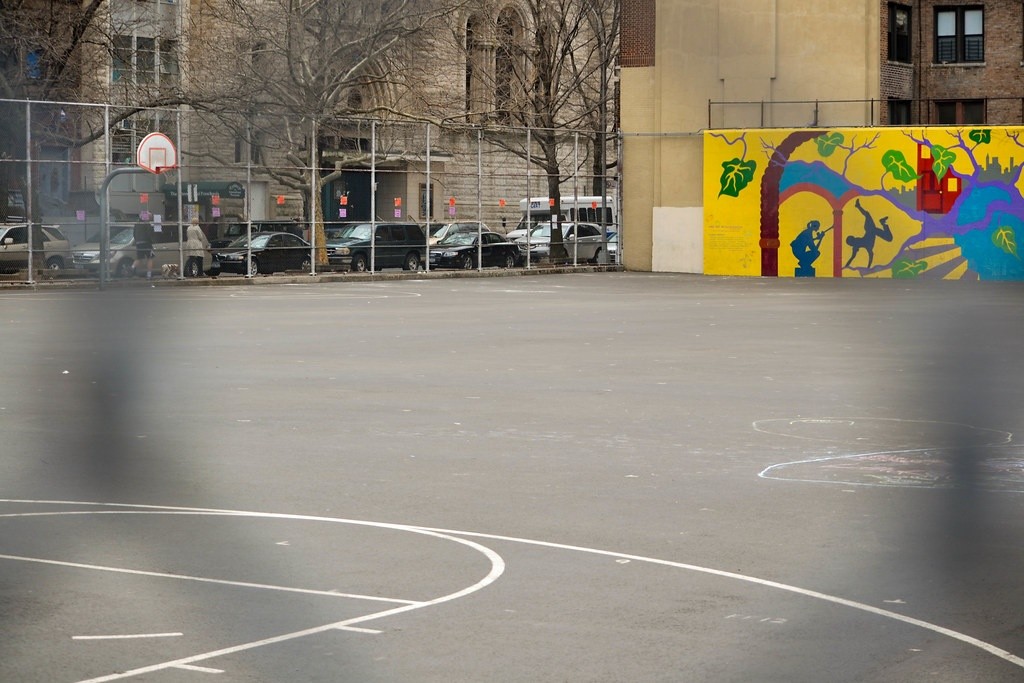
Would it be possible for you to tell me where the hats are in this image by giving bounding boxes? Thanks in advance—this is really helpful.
[140,211,151,220]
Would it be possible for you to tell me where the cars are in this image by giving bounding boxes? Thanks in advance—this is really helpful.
[421,232,519,270]
[421,220,490,246]
[513,223,602,264]
[213,231,312,277]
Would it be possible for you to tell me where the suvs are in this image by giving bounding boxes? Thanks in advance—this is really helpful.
[69,224,213,279]
[326,223,428,272]
[0,223,71,274]
[212,219,305,250]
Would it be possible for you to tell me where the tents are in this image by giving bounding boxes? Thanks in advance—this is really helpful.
[160,181,245,234]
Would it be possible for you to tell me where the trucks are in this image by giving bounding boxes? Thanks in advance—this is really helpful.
[514,197,617,252]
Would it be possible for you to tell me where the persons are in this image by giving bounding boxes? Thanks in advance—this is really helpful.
[186,218,210,276]
[129,212,156,280]
[294,219,303,238]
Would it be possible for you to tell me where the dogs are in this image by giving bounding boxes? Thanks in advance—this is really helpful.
[203,252,221,279]
[161,263,179,279]
